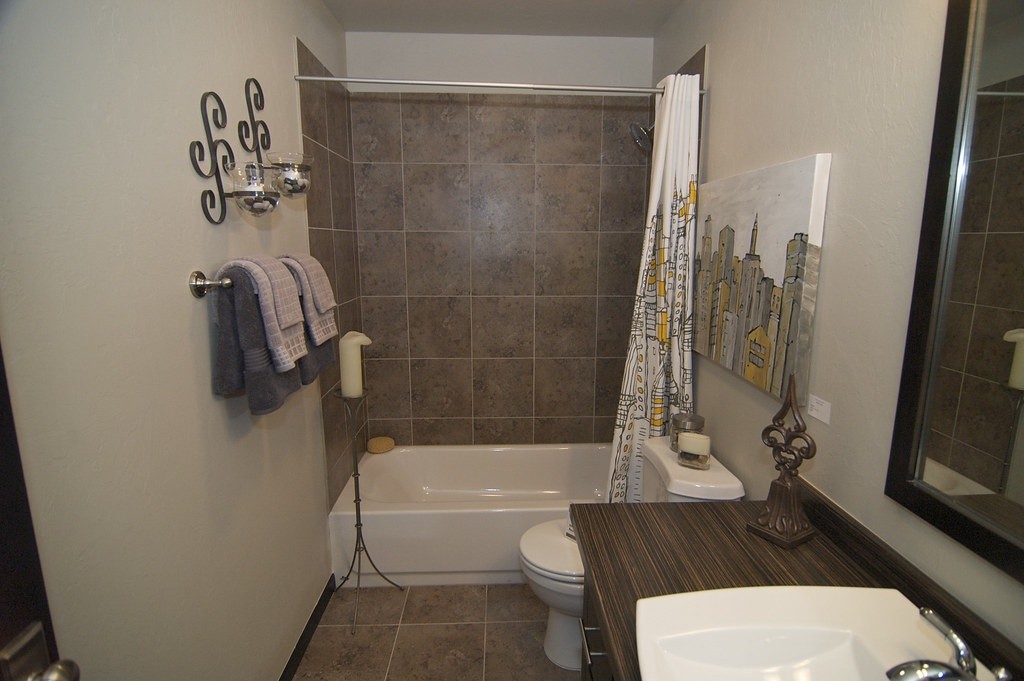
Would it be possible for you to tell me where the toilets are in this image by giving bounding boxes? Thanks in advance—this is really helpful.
[518,433,746,673]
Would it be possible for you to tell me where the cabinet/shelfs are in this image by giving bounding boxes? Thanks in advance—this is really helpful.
[579,572,615,681]
[189,77,314,225]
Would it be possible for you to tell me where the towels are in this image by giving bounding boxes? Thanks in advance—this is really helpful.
[207,251,341,418]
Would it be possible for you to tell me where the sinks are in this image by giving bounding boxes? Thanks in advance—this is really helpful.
[632,580,1011,681]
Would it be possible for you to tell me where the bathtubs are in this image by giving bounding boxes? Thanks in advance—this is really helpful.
[328,440,615,586]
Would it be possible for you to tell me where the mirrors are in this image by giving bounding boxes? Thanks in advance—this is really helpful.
[885,0,1024,583]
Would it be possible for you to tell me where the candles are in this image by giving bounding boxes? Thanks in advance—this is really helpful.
[1003,328,1024,391]
[677,431,710,470]
[339,331,372,397]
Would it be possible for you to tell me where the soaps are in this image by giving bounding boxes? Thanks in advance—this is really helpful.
[366,436,396,454]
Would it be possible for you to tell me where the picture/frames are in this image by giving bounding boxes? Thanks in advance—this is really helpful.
[692,151,832,407]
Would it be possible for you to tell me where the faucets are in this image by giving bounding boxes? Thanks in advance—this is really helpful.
[885,602,979,681]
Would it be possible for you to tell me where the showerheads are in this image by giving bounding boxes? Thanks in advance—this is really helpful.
[629,120,655,155]
